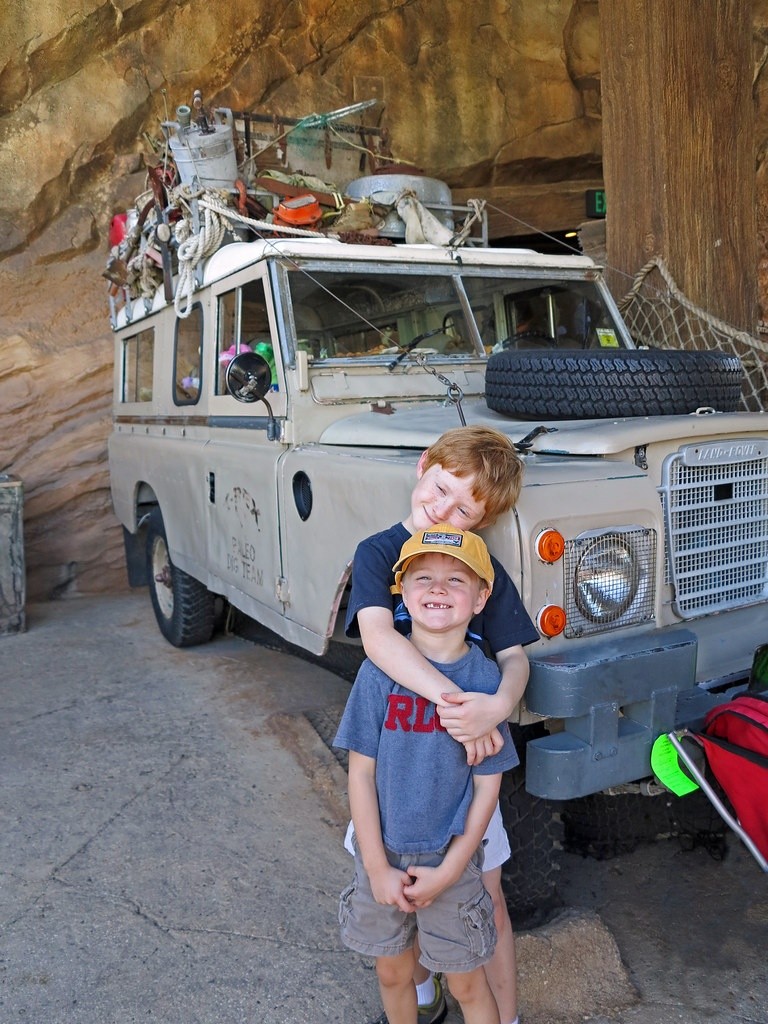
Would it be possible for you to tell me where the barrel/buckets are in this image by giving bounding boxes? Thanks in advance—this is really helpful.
[346,174,455,238]
[110,125,249,250]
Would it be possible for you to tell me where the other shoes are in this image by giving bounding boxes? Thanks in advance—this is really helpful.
[369,969,451,1024]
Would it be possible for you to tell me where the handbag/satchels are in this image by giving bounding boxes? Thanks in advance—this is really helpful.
[690,694,768,862]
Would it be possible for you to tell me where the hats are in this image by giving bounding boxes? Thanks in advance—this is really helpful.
[395,522,496,591]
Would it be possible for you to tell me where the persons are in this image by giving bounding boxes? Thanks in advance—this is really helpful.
[343,425,542,1024]
[332,521,521,1024]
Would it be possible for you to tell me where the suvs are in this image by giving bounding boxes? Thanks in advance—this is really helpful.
[108,88,768,918]
